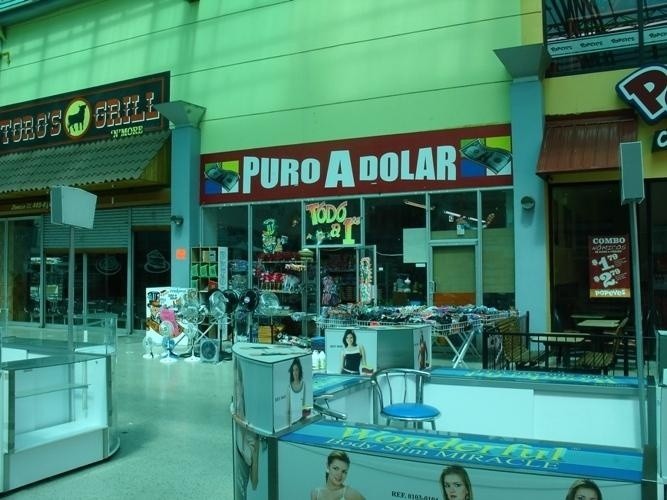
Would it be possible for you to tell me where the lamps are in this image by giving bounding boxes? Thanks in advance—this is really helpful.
[171,216,184,227]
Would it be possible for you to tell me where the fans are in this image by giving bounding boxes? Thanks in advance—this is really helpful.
[142,287,280,364]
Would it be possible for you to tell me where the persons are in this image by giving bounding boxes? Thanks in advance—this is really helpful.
[337,328,367,376]
[283,358,306,428]
[307,449,366,500]
[233,423,262,500]
[232,358,247,421]
[438,463,474,500]
[415,329,428,373]
[565,479,602,500]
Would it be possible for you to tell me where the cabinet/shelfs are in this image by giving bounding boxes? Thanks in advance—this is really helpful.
[189,247,229,341]
[230,258,320,338]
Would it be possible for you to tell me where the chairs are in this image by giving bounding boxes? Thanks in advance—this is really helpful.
[574,316,629,376]
[496,314,546,370]
[370,368,442,430]
